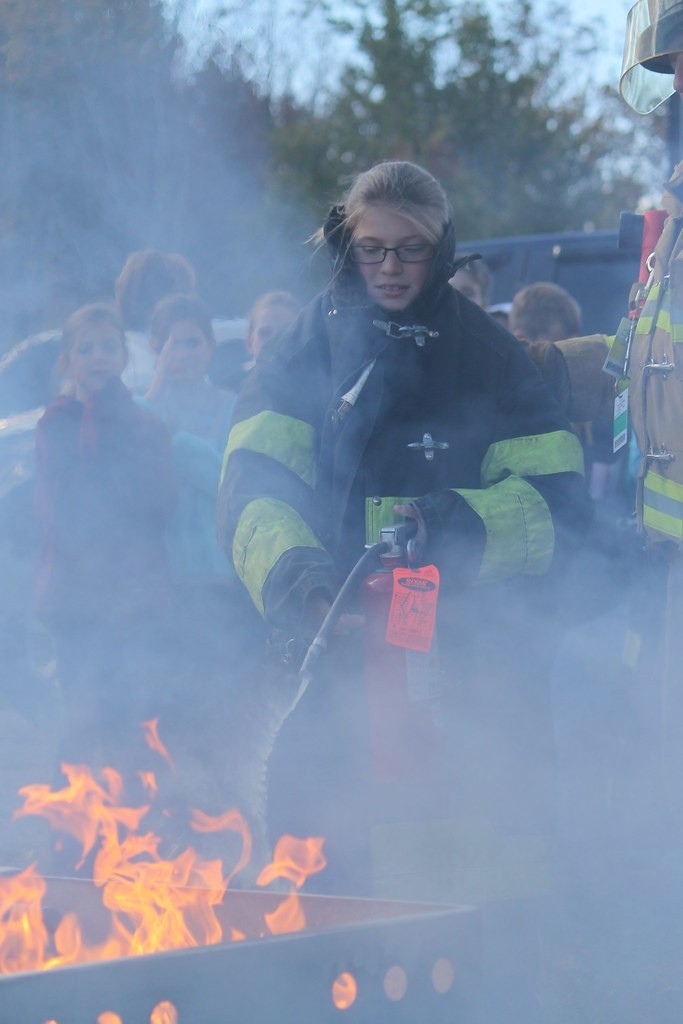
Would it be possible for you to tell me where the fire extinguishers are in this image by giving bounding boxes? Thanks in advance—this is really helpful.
[295,509,438,787]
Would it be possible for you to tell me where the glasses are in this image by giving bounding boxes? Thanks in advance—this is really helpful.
[345,239,439,265]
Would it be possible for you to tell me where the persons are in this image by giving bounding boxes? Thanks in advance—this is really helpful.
[213,159,597,910]
[519,1,683,1024]
[25,251,613,872]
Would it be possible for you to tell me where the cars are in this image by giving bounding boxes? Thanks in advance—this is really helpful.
[2,314,257,505]
